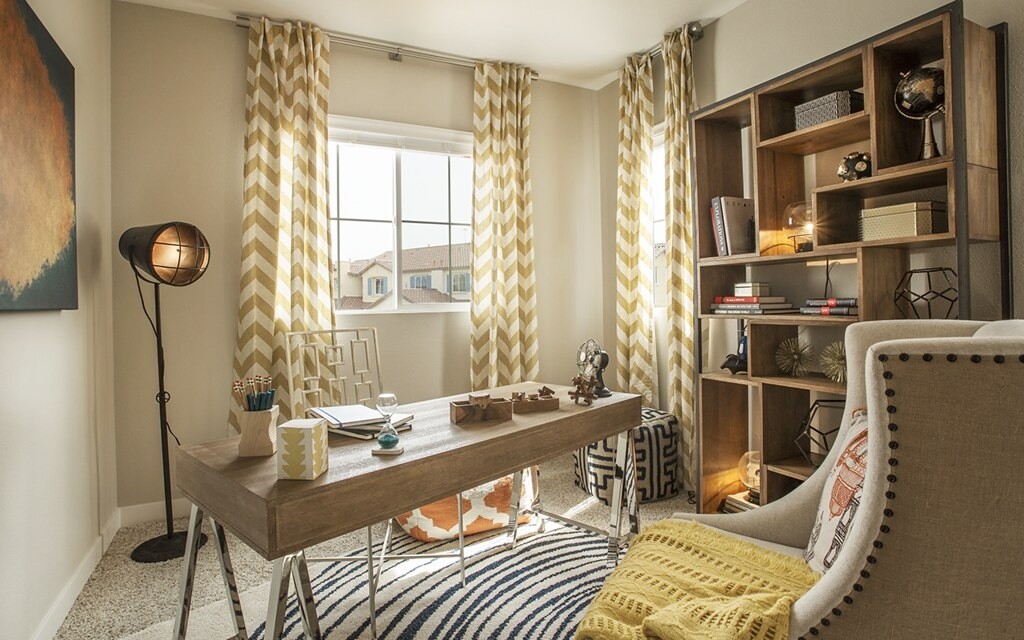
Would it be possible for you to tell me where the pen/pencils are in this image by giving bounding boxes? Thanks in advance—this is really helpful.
[233,375,275,411]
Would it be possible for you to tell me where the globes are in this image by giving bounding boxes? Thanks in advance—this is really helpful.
[894,67,946,158]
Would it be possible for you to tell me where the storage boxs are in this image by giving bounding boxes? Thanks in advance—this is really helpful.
[277,419,329,480]
[857,201,948,242]
[792,91,864,131]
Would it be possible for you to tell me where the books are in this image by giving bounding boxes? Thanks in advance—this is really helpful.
[305,402,415,441]
[708,281,859,318]
[709,195,758,256]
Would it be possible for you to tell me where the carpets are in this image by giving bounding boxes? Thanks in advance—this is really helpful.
[119,512,630,640]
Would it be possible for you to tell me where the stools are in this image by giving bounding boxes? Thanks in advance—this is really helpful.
[394,462,536,542]
[575,407,681,507]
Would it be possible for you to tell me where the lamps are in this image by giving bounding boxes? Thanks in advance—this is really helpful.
[119,221,208,563]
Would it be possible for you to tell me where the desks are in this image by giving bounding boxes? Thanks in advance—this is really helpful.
[173,380,642,640]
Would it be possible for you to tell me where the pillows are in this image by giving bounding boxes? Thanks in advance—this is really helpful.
[805,411,869,578]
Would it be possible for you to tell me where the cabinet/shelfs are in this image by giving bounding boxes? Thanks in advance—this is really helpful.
[696,1,1010,515]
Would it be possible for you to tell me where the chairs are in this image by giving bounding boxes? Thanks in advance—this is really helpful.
[286,328,465,640]
[579,317,1024,640]
